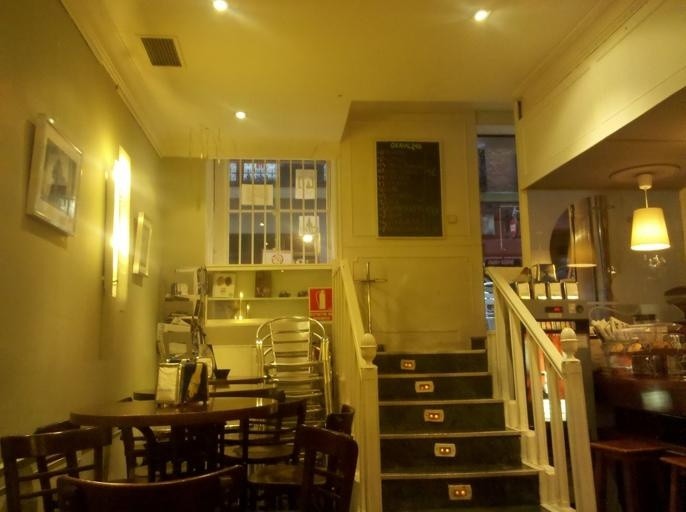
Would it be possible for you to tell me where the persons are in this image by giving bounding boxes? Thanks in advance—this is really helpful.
[212,276,224,297]
[222,277,234,298]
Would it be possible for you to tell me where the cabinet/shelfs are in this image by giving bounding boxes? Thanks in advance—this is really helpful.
[156,263,335,328]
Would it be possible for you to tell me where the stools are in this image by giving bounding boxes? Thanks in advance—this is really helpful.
[589,433,686,512]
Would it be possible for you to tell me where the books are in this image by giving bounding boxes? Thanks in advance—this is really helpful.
[210,272,235,299]
[252,271,272,298]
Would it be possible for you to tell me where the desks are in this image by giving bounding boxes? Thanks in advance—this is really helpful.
[131,374,274,481]
[537,326,685,418]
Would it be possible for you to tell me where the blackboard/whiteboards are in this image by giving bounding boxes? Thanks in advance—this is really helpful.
[373,138,445,240]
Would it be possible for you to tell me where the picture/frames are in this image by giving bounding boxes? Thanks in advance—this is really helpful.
[26,113,83,235]
[132,211,153,277]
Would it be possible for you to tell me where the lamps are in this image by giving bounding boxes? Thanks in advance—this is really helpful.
[630,173,672,251]
[515,263,580,301]
[568,197,597,268]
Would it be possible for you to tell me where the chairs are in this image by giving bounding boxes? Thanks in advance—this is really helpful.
[255,313,336,468]
[1,387,358,511]
